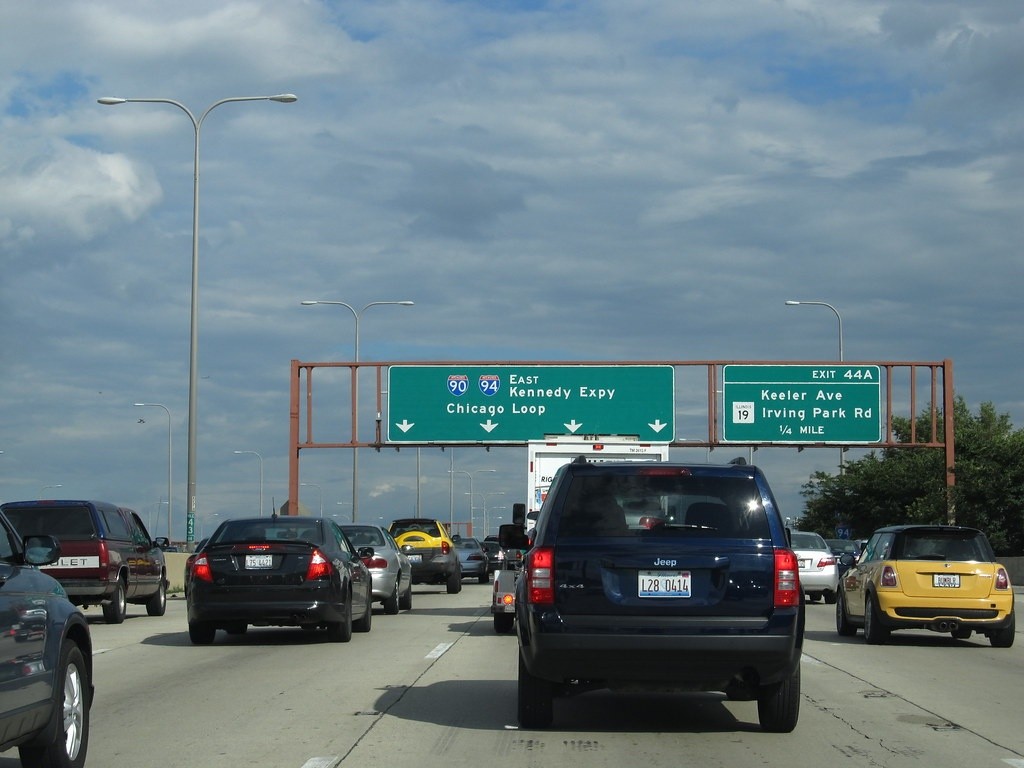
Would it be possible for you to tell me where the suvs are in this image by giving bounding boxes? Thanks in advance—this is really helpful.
[499,455,806,732]
[388,518,461,593]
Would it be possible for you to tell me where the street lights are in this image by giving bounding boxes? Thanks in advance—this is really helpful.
[234,451,262,517]
[133,403,172,546]
[448,470,506,538]
[301,300,414,524]
[785,301,845,476]
[98,94,298,552]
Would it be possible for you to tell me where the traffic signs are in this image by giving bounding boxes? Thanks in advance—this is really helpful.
[387,365,675,444]
[723,365,882,444]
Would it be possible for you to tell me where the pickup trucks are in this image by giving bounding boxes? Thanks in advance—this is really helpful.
[0,499,170,623]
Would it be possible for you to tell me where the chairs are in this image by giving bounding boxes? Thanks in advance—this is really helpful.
[684,503,740,532]
[238,526,267,543]
[569,503,629,536]
[353,534,373,545]
[302,529,321,545]
[943,538,969,558]
[430,528,438,535]
[396,528,406,538]
[465,543,473,548]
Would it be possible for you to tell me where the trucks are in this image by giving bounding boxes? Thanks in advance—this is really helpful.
[527,433,670,545]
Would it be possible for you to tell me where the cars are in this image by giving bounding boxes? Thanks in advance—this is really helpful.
[184,516,412,645]
[791,532,868,604]
[836,525,1016,648]
[452,535,523,584]
[491,528,535,633]
[0,510,95,768]
